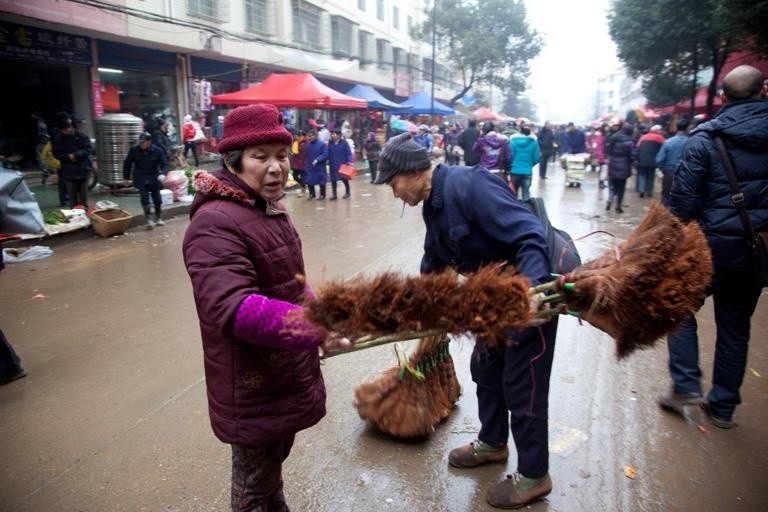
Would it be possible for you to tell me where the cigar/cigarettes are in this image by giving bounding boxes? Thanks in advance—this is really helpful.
[400,201,406,218]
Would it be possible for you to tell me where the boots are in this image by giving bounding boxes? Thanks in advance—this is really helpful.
[598,181,608,189]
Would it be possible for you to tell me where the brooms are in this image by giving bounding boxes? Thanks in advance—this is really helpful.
[354,264,462,436]
[276,199,714,362]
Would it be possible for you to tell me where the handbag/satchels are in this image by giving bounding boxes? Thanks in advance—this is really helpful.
[519,196,584,288]
[451,144,465,158]
[751,228,767,289]
[599,164,610,181]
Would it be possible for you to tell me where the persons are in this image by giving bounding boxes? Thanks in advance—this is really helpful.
[122,132,170,229]
[141,108,225,202]
[33,111,101,211]
[0,330,27,388]
[654,63,767,430]
[181,102,355,511]
[371,129,583,509]
[605,123,639,214]
[283,108,709,201]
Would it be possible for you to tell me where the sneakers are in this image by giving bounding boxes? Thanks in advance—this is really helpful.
[614,208,624,213]
[1,368,27,387]
[330,195,337,200]
[485,467,552,509]
[307,194,316,200]
[606,201,611,210]
[646,194,652,197]
[639,191,644,198]
[297,190,305,197]
[540,176,548,179]
[448,439,509,468]
[145,219,155,229]
[316,195,325,200]
[301,186,307,191]
[342,193,351,198]
[156,218,165,225]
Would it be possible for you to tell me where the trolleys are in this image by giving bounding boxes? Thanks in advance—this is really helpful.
[558,150,591,188]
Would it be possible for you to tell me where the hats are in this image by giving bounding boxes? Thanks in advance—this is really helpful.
[217,103,294,153]
[184,115,193,123]
[137,131,153,144]
[330,127,342,135]
[374,131,433,185]
[419,124,432,132]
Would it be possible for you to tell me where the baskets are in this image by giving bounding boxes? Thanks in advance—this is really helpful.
[90,207,134,238]
[337,163,356,181]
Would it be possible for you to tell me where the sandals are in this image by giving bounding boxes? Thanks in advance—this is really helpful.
[699,397,734,429]
[655,384,708,410]
